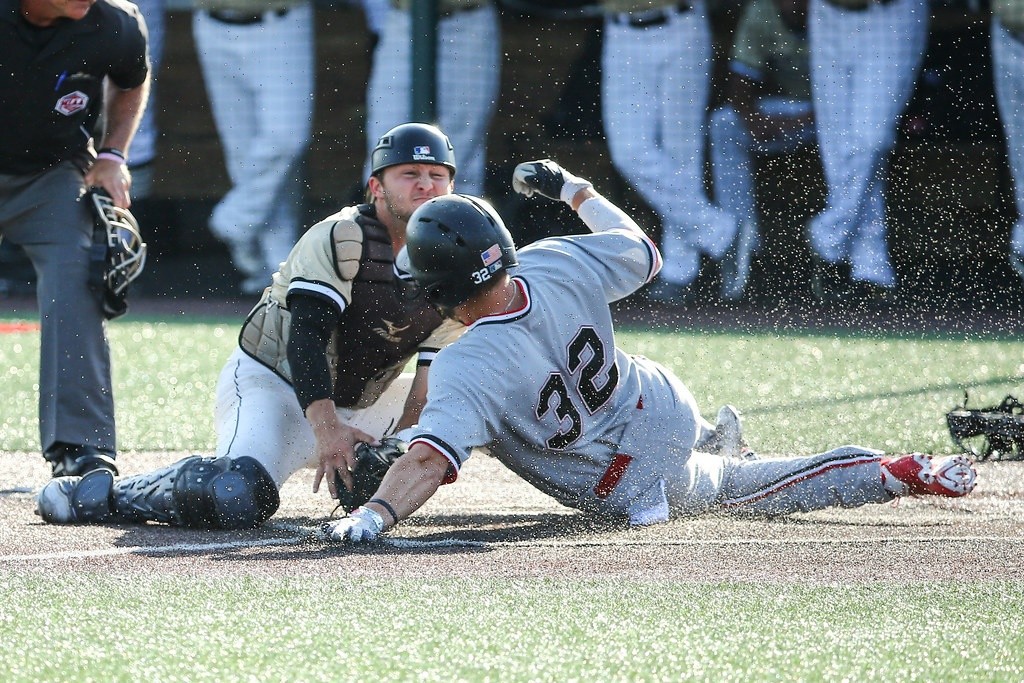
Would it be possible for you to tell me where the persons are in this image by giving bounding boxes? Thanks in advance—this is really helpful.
[601,0,754,304]
[364,0,502,203]
[805,0,931,314]
[0,0,151,477]
[39,122,978,545]
[191,0,315,295]
[96,0,166,294]
[990,0,1024,300]
[709,0,819,242]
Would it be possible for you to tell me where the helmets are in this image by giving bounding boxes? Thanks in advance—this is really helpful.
[370,122,458,177]
[396,194,519,316]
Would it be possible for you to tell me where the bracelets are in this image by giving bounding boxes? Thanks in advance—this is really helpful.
[98,148,127,164]
[369,499,398,525]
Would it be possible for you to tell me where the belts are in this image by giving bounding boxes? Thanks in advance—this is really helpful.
[628,6,692,28]
[595,398,645,499]
[208,9,288,25]
[436,4,480,21]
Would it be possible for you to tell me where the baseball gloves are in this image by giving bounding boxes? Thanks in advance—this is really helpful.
[333,437,409,514]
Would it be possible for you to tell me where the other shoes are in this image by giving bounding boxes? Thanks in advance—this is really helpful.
[720,222,753,304]
[648,280,693,308]
[207,218,259,276]
[805,227,834,310]
[844,281,897,310]
[241,270,275,295]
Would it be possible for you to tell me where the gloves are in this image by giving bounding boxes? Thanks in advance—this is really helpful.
[317,505,383,544]
[512,159,593,208]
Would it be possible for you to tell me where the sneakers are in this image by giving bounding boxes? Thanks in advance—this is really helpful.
[38,477,84,525]
[716,406,745,459]
[879,453,977,498]
[50,446,119,479]
